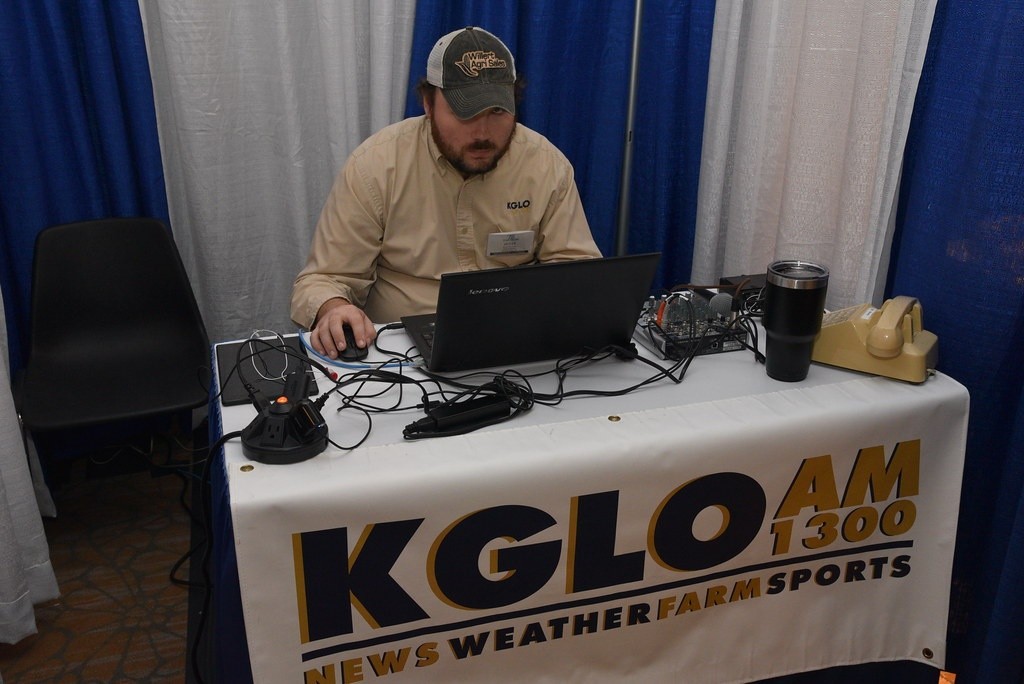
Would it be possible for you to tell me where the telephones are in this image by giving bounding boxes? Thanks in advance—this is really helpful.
[807,295,938,384]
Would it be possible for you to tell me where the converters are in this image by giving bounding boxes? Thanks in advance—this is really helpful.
[429,396,510,431]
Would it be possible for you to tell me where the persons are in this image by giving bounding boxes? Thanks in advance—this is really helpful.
[288,25,603,362]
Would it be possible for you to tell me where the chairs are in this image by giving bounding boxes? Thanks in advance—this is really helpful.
[20,215,211,476]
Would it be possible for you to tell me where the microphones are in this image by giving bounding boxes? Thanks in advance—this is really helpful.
[692,287,733,316]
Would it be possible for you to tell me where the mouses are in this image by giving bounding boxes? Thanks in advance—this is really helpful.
[333,325,368,362]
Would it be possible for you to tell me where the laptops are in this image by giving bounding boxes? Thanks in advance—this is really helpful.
[400,252,663,373]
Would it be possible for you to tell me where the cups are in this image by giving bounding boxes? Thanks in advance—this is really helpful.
[764,259,830,382]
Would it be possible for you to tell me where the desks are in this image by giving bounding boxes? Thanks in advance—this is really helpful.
[212,283,971,684]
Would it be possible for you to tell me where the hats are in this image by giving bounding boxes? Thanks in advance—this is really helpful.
[427,26,515,120]
